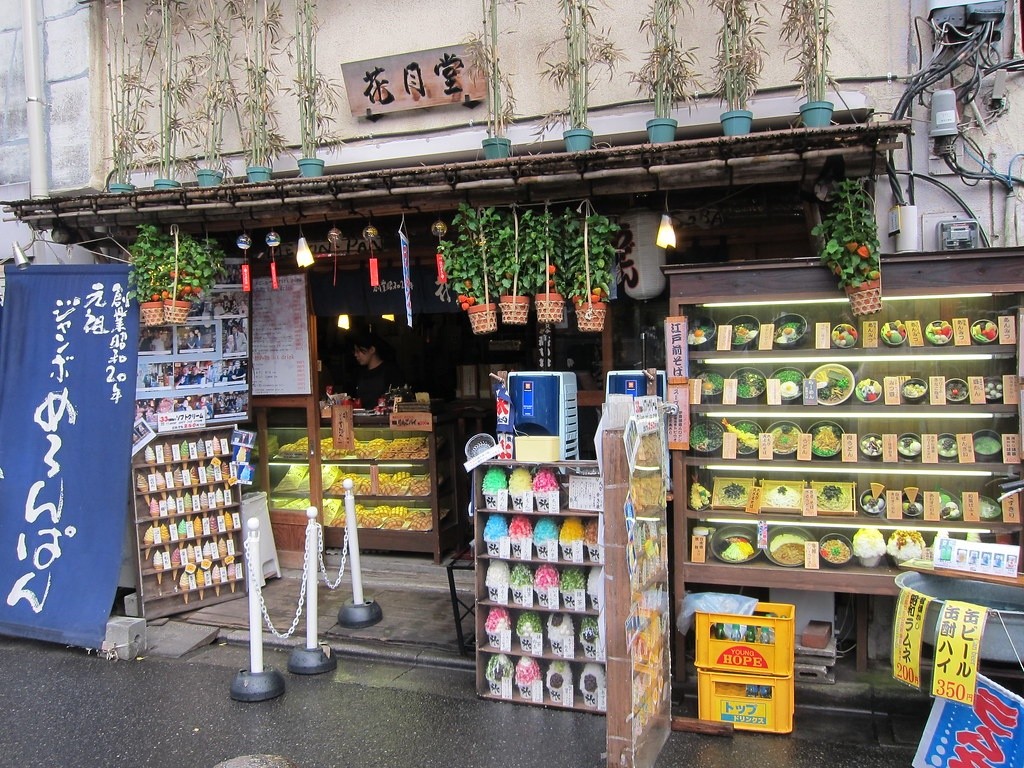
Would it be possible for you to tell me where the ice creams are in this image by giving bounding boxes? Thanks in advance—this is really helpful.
[480,466,605,707]
[137,436,235,603]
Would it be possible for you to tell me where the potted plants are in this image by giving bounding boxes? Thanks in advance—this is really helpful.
[126,223,226,327]
[708,0,771,135]
[94,0,347,197]
[468,0,528,159]
[782,0,840,128]
[533,0,632,155]
[438,199,622,335]
[639,0,707,144]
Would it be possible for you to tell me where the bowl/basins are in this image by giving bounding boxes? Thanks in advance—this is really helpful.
[924,320,953,344]
[881,322,907,348]
[809,364,855,406]
[768,367,807,405]
[983,376,1004,404]
[692,369,726,404]
[831,324,858,347]
[726,315,760,350]
[897,432,922,459]
[970,319,998,345]
[773,313,807,350]
[945,378,969,404]
[972,429,1002,462]
[687,314,716,351]
[901,378,928,405]
[937,432,958,461]
[895,571,1024,662]
[729,366,768,404]
[689,420,883,460]
[707,488,1002,569]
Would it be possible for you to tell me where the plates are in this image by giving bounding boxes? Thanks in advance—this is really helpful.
[355,475,433,531]
[272,448,383,460]
[856,380,882,404]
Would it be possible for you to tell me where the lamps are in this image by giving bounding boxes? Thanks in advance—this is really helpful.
[297,224,316,267]
[656,190,676,249]
[338,314,350,330]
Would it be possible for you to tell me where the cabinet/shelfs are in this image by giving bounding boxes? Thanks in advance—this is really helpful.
[131,422,256,622]
[470,456,608,715]
[261,413,459,563]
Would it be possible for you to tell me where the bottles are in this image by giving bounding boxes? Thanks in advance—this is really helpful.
[706,527,716,560]
[692,527,709,537]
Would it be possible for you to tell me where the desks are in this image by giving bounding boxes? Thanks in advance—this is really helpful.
[449,398,497,438]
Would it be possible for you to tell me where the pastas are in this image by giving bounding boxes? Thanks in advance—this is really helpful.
[718,483,748,505]
[764,485,802,507]
[818,486,850,510]
[772,429,801,453]
[822,540,849,563]
[814,429,836,450]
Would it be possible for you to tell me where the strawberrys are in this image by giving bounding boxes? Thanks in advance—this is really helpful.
[458,265,601,311]
[151,270,201,300]
[834,242,881,280]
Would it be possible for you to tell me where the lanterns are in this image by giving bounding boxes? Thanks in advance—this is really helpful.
[619,205,667,300]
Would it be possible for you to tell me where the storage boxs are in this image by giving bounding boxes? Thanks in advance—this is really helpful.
[693,601,795,677]
[697,667,796,734]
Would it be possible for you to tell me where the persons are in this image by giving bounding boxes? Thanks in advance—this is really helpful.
[214,394,243,413]
[196,395,212,419]
[174,365,208,388]
[177,399,188,411]
[151,327,166,351]
[187,327,204,348]
[139,328,152,351]
[228,361,246,381]
[219,369,229,381]
[214,294,240,316]
[336,332,406,409]
[144,369,158,387]
[226,324,247,353]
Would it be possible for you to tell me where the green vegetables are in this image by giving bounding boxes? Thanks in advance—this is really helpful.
[837,378,849,388]
[699,326,709,331]
[708,374,724,388]
[690,426,706,444]
[785,323,798,328]
[734,335,745,344]
[776,370,802,385]
[737,385,752,399]
[813,447,835,456]
[737,424,753,433]
[831,547,839,555]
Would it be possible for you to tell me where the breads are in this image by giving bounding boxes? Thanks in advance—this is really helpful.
[280,437,446,529]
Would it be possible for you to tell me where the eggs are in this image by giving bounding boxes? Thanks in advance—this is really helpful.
[701,382,715,391]
[781,381,798,397]
